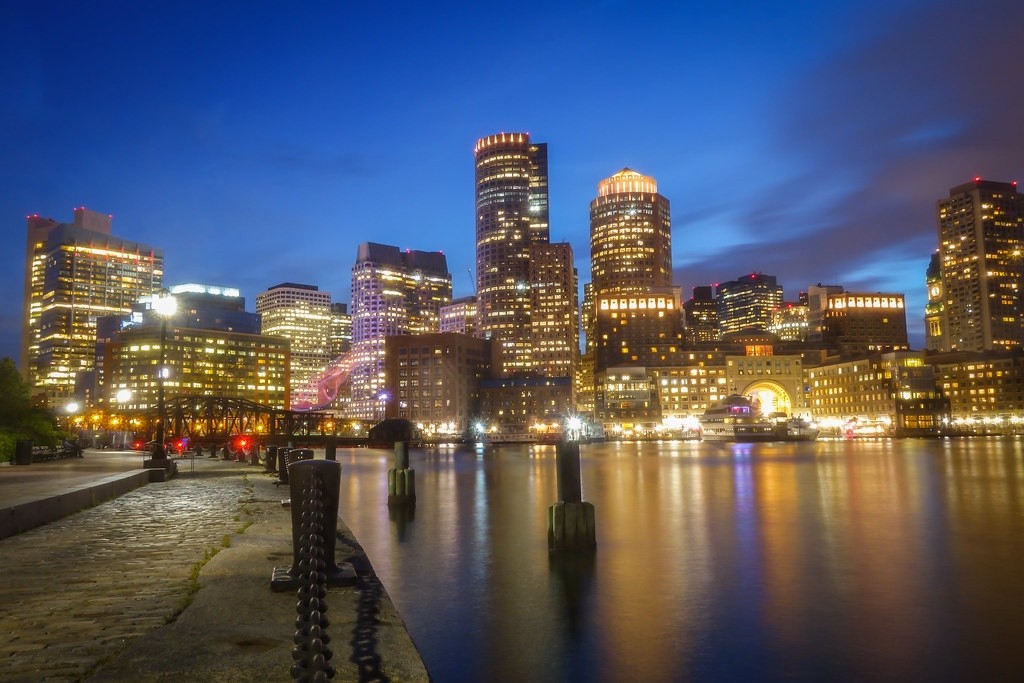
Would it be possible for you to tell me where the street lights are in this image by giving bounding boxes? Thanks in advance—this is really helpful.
[151,293,178,460]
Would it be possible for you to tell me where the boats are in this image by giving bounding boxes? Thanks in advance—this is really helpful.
[731,408,820,443]
